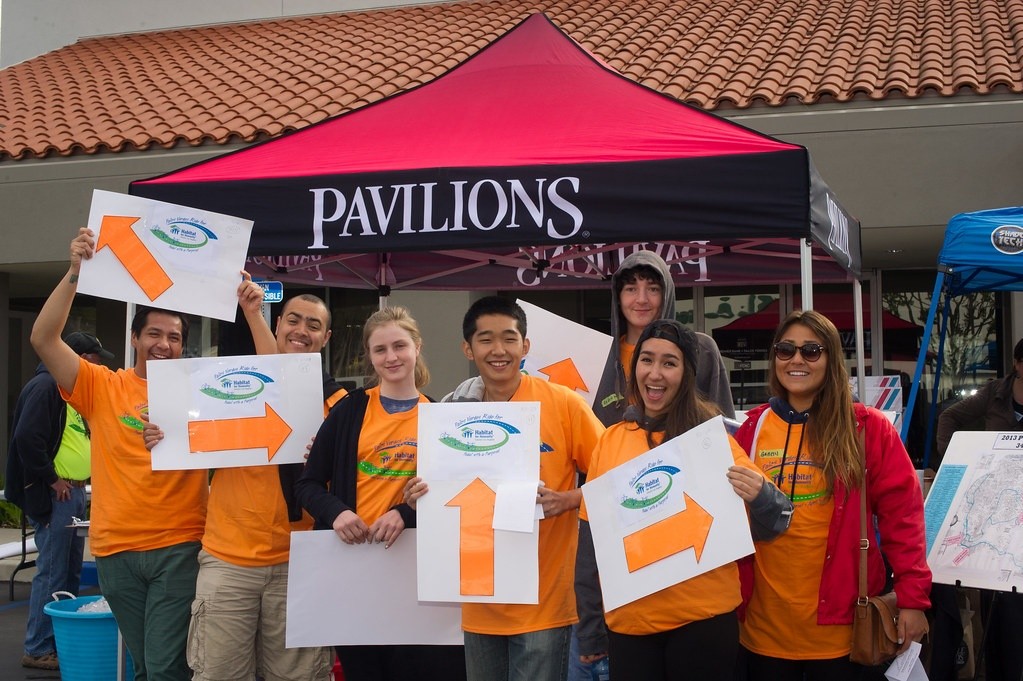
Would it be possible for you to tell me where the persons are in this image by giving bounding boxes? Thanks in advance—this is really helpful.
[299,307,437,670]
[587,250,735,428]
[732,309,932,680]
[571,495,575,497]
[572,318,793,681]
[139,293,348,680]
[4,331,116,670]
[936,340,1023,681]
[29,225,281,679]
[401,295,611,680]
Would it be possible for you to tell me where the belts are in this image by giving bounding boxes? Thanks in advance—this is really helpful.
[62,478,87,487]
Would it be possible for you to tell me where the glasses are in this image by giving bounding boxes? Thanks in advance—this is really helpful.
[772,342,826,362]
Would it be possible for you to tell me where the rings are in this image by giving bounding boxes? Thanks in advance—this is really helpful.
[62,490,65,493]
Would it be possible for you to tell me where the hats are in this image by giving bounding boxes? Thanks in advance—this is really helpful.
[64,332,115,360]
[638,319,700,377]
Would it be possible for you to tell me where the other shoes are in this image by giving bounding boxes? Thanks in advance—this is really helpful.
[22,653,59,669]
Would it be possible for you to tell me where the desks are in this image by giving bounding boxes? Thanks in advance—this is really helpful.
[0,485,91,601]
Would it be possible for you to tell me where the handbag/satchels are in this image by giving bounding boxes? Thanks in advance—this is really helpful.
[850,588,900,664]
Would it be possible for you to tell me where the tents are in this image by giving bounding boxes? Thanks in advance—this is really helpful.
[901,206,1022,448]
[710,283,923,409]
[122,12,864,411]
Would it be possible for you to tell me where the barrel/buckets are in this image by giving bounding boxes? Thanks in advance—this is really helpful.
[43,591,135,681]
